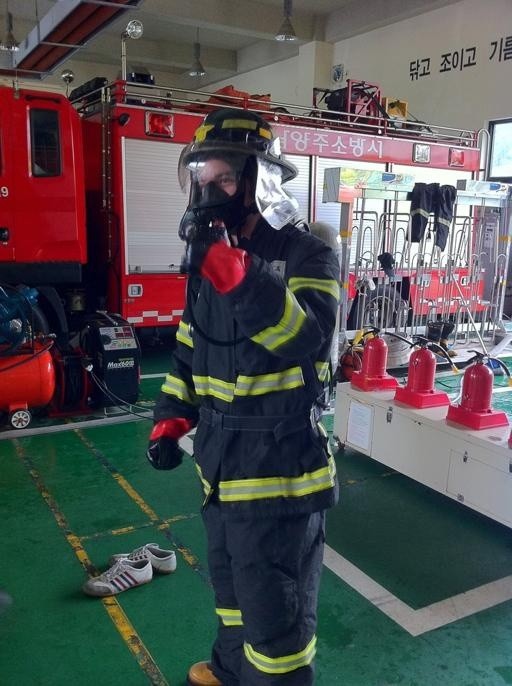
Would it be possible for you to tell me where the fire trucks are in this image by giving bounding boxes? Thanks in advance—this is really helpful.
[0,19,512,339]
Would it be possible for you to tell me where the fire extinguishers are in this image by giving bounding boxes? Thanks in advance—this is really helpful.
[350,325,512,431]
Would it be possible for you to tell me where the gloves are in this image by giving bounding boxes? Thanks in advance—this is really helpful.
[180,187,229,246]
[149,425,184,469]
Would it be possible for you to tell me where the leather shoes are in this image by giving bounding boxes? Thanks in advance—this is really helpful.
[187,661,223,686]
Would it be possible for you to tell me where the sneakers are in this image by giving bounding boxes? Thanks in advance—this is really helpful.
[82,543,176,598]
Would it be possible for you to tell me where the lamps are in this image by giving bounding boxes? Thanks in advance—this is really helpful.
[1,1,19,52]
[274,0,298,41]
[189,28,206,77]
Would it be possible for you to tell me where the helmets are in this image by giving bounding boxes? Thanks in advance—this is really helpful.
[182,109,298,184]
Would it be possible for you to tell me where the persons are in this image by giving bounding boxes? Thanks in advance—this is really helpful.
[146,108,340,686]
